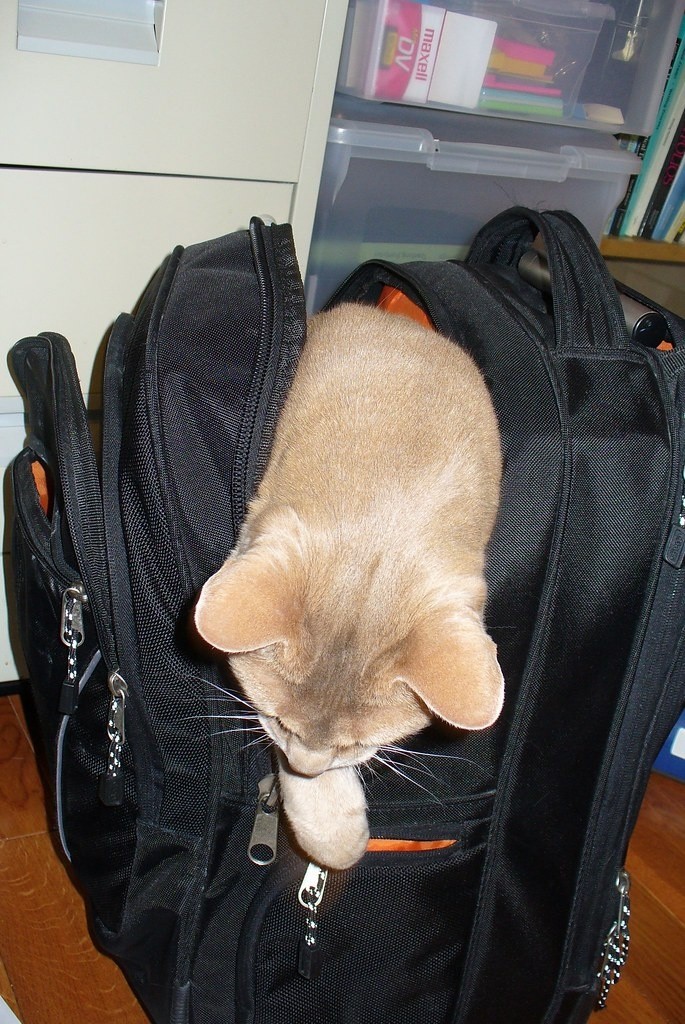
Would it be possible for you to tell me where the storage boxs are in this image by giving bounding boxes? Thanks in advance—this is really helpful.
[304,0,685,315]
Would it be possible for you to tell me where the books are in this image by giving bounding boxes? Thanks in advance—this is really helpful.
[604,11,685,247]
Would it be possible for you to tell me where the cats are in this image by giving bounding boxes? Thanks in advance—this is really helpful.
[195,305,506,871]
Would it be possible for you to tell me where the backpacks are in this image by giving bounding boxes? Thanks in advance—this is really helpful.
[3,206,685,1024]
